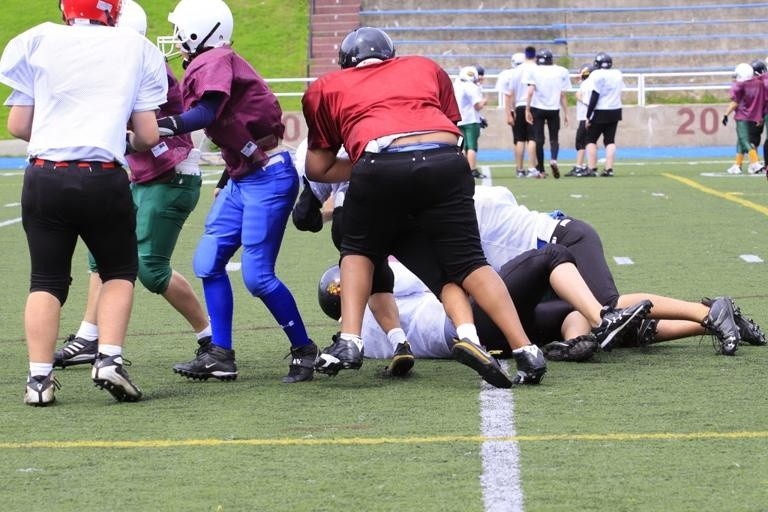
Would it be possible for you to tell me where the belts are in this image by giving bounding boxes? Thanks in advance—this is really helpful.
[35,159,115,169]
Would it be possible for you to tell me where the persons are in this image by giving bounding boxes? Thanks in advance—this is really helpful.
[300,26,548,385]
[0,0,170,407]
[51,0,217,370]
[155,0,321,383]
[289,132,767,363]
[720,59,768,177]
[450,45,625,180]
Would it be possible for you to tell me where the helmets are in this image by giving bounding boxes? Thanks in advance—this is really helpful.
[459,65,484,83]
[511,52,526,68]
[734,60,767,82]
[535,48,552,65]
[580,53,612,80]
[296,137,349,178]
[338,27,395,69]
[318,264,341,323]
[59,0,147,36]
[157,0,234,62]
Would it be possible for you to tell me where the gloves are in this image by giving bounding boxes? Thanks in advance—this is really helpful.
[723,116,727,125]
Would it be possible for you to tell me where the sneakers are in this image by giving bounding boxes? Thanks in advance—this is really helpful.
[452,336,512,388]
[516,168,548,179]
[550,159,559,178]
[727,165,743,174]
[172,336,239,381]
[384,341,415,375]
[283,331,364,383]
[540,300,657,362]
[472,170,486,178]
[702,296,768,355]
[748,161,766,174]
[512,344,547,384]
[564,168,613,177]
[24,334,142,407]
